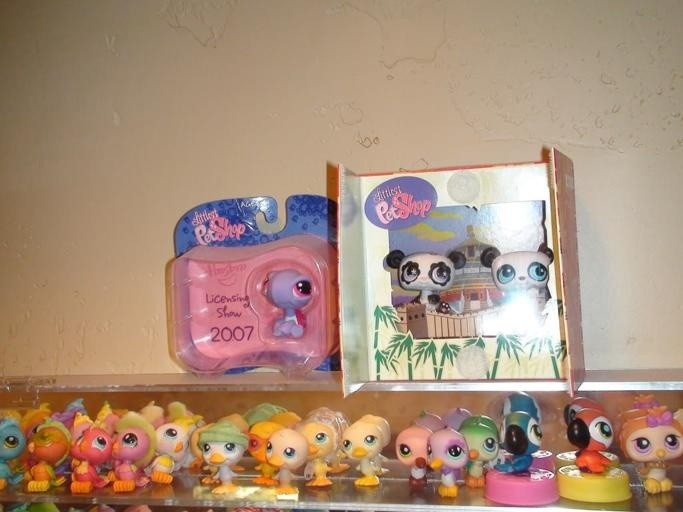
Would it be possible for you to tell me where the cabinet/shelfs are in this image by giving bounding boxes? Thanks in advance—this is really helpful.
[0,373,683,512]
